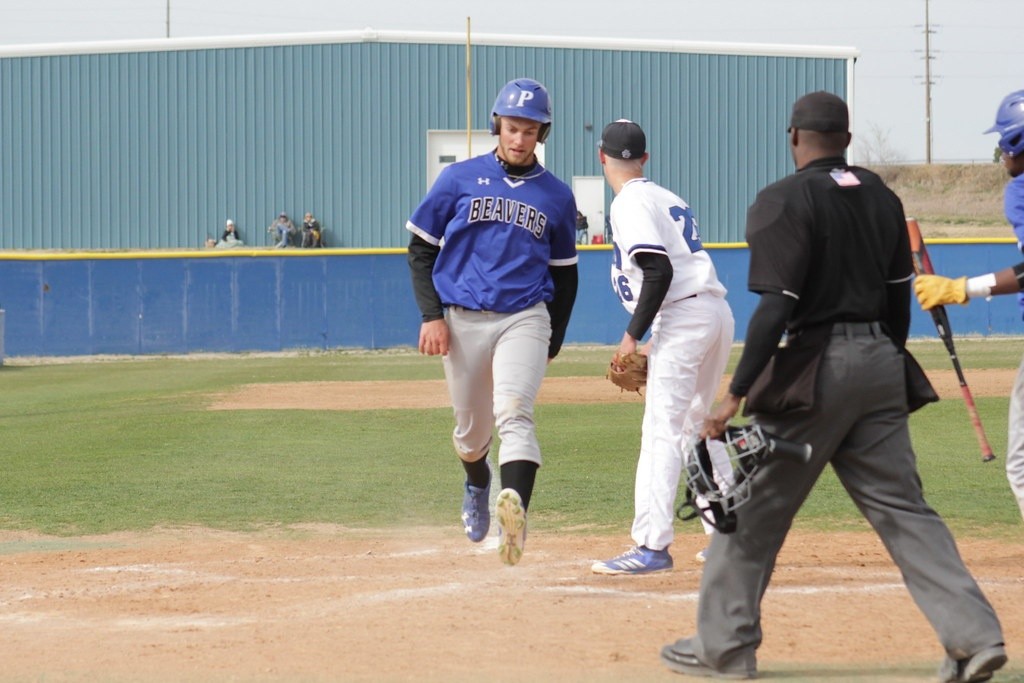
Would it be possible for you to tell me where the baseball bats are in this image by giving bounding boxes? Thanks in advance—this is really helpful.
[905,217,995,462]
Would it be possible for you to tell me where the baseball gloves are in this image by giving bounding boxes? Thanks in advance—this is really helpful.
[610,351,647,391]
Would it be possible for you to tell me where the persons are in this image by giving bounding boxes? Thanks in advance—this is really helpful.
[302,212,322,248]
[405,77,579,567]
[660,88,1008,683]
[215,219,244,247]
[589,118,736,575]
[272,213,296,247]
[913,89,1024,523]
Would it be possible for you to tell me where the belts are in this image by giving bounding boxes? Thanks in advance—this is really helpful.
[446,304,483,312]
[830,319,881,337]
[674,294,697,302]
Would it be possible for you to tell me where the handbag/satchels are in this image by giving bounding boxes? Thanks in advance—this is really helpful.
[742,347,821,415]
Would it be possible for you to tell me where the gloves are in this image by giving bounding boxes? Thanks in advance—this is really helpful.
[911,274,970,311]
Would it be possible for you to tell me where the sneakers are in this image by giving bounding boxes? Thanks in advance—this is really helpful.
[493,488,528,567]
[461,457,492,543]
[592,546,674,574]
[696,549,708,563]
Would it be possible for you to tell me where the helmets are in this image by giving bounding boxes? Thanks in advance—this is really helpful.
[489,78,552,144]
[983,89,1024,159]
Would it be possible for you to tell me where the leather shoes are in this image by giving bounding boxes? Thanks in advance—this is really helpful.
[941,644,1008,683]
[661,642,758,678]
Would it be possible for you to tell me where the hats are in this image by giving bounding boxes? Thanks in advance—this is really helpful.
[226,219,234,226]
[787,90,849,133]
[598,118,645,161]
